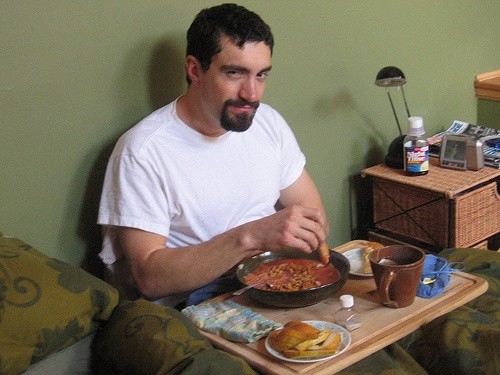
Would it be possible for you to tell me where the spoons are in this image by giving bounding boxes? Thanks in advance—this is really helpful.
[232,283,278,296]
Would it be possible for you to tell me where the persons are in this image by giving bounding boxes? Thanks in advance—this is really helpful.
[96,3,330,309]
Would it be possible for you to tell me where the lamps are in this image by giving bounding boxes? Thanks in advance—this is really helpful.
[373,66,412,168]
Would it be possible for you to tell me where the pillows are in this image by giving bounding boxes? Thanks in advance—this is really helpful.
[0,232,118,375]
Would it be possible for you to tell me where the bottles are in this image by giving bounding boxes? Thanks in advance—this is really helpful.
[403,116,429,176]
[334,295,363,331]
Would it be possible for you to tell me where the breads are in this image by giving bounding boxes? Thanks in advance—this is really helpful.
[364,242,388,273]
[269,320,341,358]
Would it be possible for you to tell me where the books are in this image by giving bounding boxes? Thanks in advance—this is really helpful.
[424,118,500,168]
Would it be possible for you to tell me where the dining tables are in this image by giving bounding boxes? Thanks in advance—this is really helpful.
[182,240,489,375]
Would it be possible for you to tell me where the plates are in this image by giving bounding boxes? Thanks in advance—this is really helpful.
[264,320,351,362]
[342,248,374,276]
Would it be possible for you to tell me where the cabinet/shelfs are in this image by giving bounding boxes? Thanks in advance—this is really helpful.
[361,155,500,251]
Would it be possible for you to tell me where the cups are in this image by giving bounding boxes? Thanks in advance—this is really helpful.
[368,245,425,309]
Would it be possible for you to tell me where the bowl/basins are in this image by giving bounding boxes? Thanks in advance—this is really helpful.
[236,249,350,308]
